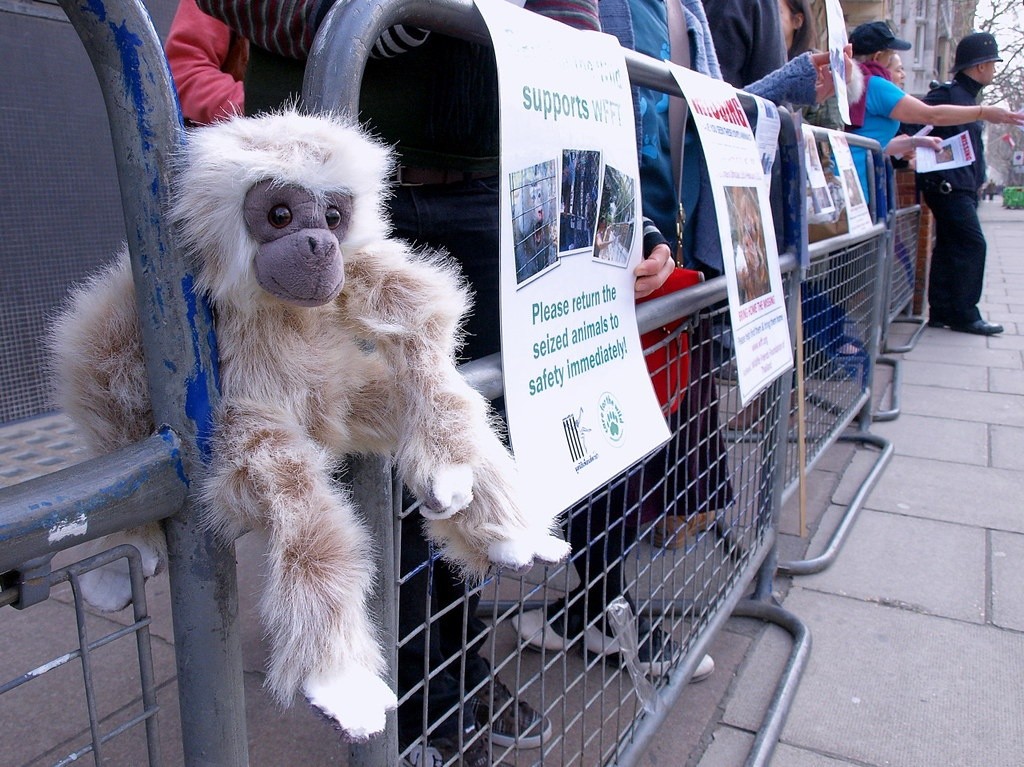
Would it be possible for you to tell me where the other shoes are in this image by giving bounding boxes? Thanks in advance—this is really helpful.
[726,390,800,432]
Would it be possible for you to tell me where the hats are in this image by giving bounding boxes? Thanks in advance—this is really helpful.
[849,22,912,55]
[948,32,1003,74]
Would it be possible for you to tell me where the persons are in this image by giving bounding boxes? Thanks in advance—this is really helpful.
[164,0,1024,765]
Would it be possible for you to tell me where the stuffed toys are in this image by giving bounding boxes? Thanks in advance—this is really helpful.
[49,108,568,737]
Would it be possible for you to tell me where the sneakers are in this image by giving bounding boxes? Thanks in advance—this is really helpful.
[466,657,553,748]
[398,721,513,766]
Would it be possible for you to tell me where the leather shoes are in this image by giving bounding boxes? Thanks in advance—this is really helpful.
[929,314,1004,335]
[510,598,585,653]
[656,511,717,549]
[583,616,716,685]
